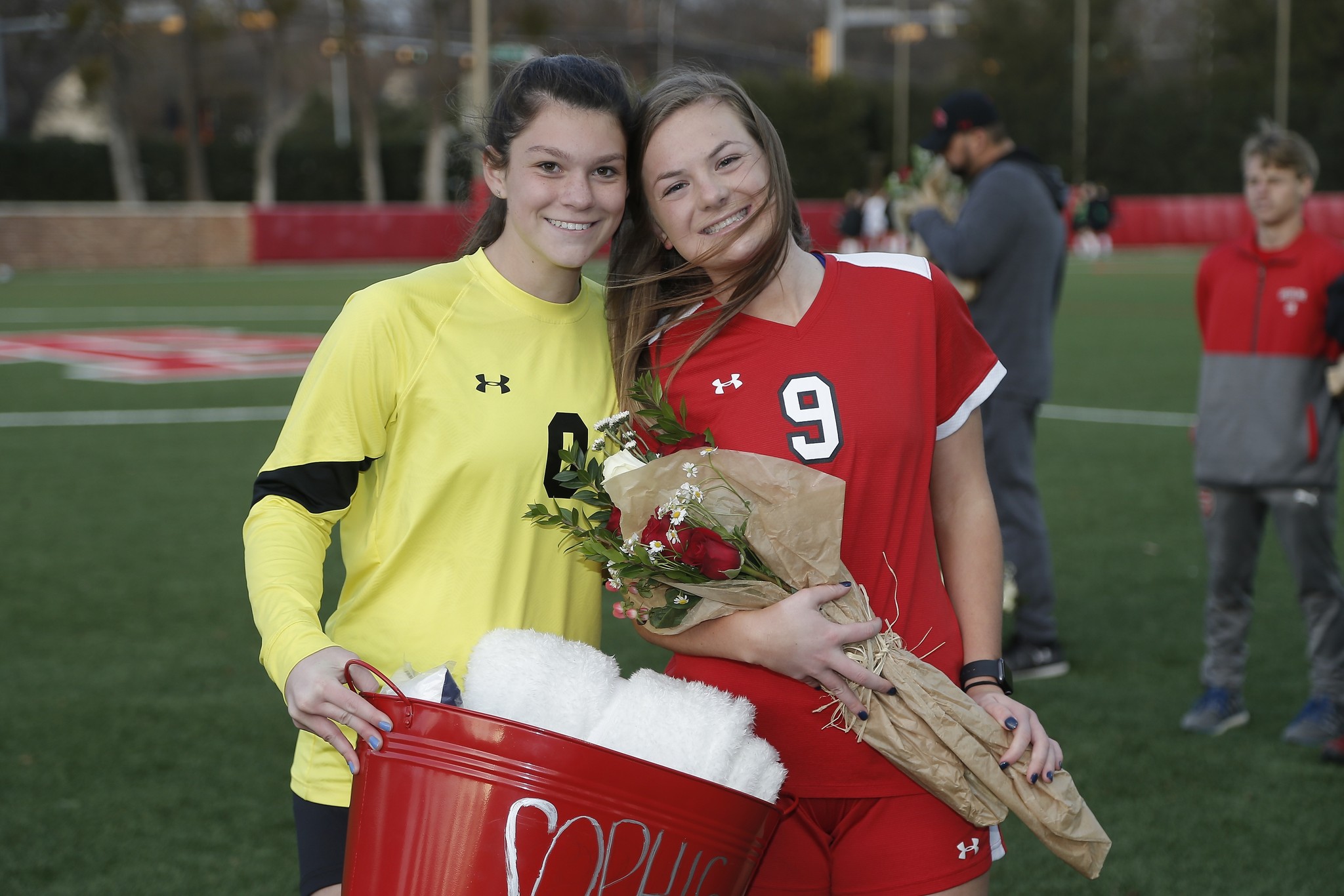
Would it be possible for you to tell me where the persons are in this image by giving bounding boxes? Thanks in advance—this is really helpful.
[905,93,1070,684]
[1181,120,1341,739]
[834,153,1115,254]
[243,53,611,894]
[608,74,1065,894]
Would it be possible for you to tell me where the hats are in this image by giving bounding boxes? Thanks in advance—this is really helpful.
[916,90,998,151]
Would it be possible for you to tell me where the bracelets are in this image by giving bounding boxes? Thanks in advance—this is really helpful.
[964,681,1001,694]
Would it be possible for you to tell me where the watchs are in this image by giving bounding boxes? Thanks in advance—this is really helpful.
[960,658,1015,696]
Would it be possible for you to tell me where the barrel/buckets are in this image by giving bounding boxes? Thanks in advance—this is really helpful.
[337,655,801,896]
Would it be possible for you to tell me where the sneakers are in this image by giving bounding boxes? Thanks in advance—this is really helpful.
[1283,699,1344,741]
[1001,631,1071,683]
[1179,685,1251,736]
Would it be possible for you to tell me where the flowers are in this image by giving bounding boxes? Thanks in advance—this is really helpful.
[523,364,1115,883]
